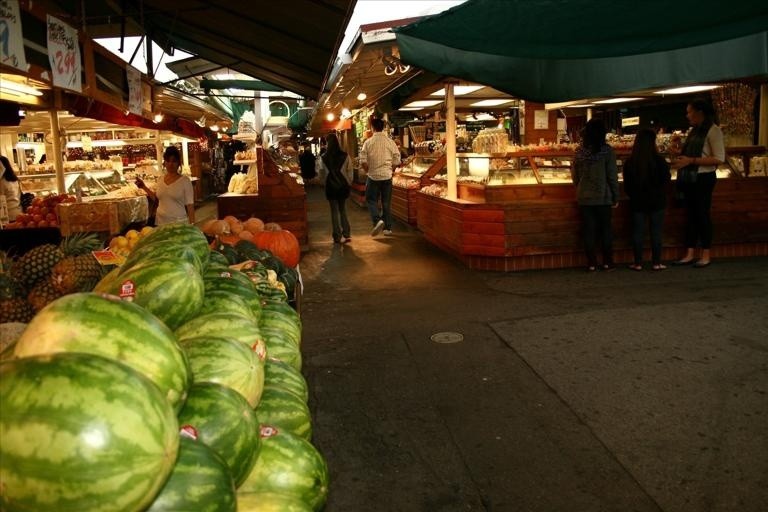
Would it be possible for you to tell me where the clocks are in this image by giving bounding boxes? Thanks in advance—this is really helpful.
[45,133,52,144]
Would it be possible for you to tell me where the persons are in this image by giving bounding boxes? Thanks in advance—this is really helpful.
[0,155,24,223]
[668,99,726,268]
[360,118,402,237]
[298,144,327,186]
[622,127,669,270]
[570,118,619,273]
[132,146,195,226]
[319,132,355,245]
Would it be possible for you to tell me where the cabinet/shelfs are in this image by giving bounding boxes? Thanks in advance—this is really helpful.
[12,132,44,142]
[67,129,154,142]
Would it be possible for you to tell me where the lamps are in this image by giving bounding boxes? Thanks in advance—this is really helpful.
[331,82,366,121]
[369,53,410,75]
[194,114,206,127]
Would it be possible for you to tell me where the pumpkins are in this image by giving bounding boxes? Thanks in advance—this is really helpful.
[253,224,300,268]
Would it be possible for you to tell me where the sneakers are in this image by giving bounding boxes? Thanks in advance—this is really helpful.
[370,219,384,237]
[587,256,712,272]
[383,229,394,236]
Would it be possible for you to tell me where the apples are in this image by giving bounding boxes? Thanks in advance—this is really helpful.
[1,193,75,229]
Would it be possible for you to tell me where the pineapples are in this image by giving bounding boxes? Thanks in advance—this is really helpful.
[2,230,118,325]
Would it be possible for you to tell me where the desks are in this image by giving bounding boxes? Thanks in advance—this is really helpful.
[61,176,199,236]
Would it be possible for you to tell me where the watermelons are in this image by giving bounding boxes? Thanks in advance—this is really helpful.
[180,335,265,411]
[145,436,238,511]
[12,293,192,417]
[93,225,264,363]
[236,299,326,510]
[176,381,263,491]
[1,351,179,511]
